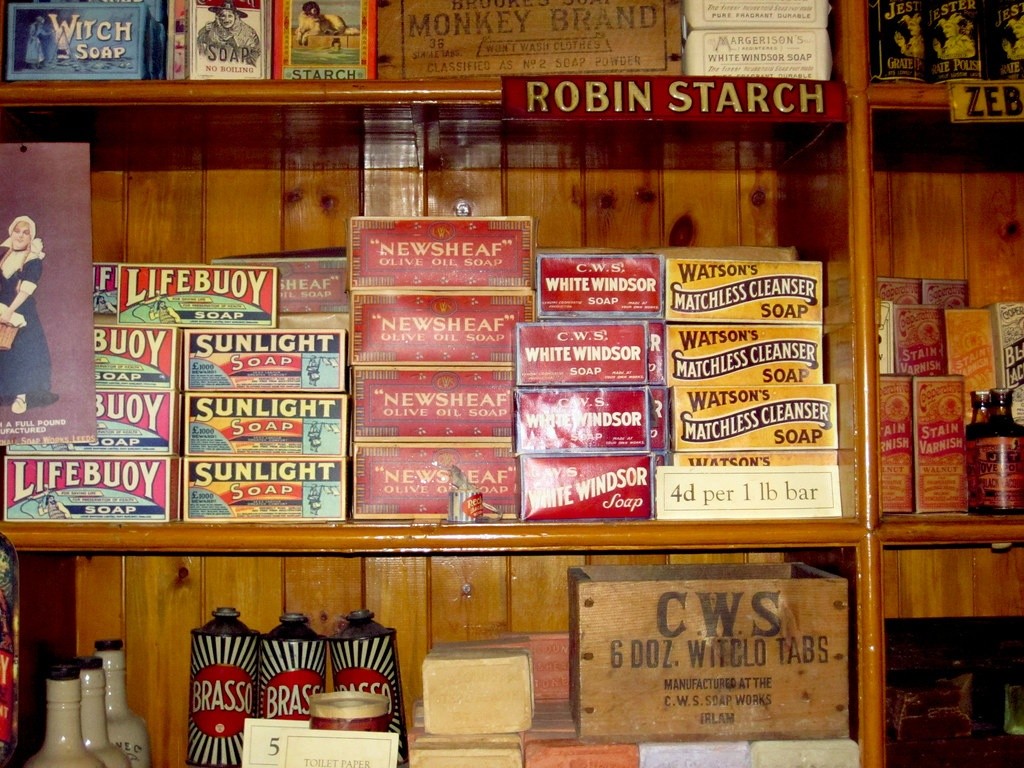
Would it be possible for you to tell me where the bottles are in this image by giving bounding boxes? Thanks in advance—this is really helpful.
[966,391,992,509]
[187,607,259,768]
[329,609,408,765]
[977,388,1023,514]
[94,638,150,767]
[21,670,105,768]
[261,611,326,713]
[74,655,133,767]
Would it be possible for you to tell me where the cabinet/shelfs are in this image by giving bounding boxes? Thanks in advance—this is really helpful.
[0,0,1024,768]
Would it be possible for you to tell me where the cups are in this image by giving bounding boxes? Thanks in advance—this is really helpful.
[310,691,390,732]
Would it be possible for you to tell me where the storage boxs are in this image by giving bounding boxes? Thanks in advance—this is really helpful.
[566,563,850,745]
[347,290,536,366]
[5,0,836,79]
[183,328,348,394]
[351,441,519,522]
[350,365,516,443]
[183,456,349,522]
[513,252,840,524]
[871,276,1024,515]
[347,216,535,290]
[3,261,279,523]
[184,393,348,457]
[210,255,349,313]
